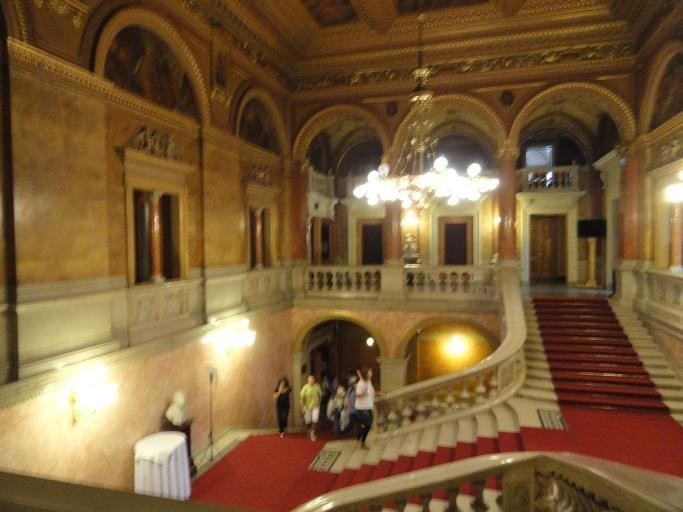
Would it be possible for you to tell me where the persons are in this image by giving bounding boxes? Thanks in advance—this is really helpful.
[354,365,382,452]
[164,391,190,427]
[298,368,362,443]
[272,376,294,439]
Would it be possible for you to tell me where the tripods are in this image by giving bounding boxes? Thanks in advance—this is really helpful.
[198,377,224,471]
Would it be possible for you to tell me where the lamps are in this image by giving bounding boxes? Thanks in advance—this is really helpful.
[349,9,504,211]
